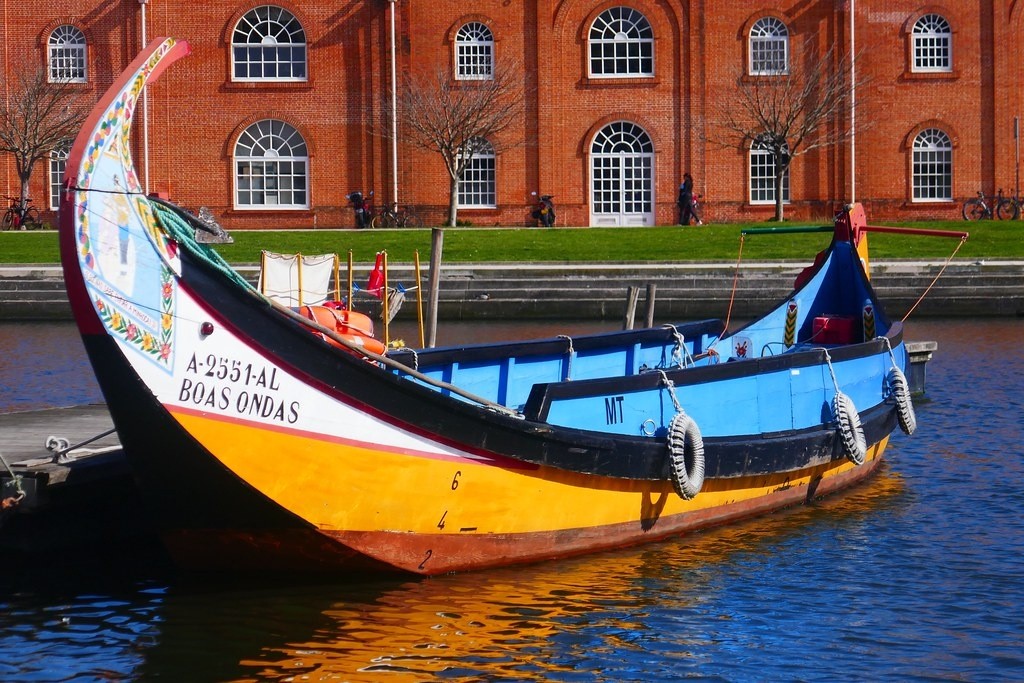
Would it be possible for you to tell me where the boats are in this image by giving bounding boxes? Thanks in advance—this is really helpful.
[57,35,969,581]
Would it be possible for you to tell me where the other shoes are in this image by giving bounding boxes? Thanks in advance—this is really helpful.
[696,221,703,226]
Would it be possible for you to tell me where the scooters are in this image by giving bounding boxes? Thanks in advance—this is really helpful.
[529,190,557,227]
[343,190,376,229]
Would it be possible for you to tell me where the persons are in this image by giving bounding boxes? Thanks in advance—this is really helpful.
[673,173,702,227]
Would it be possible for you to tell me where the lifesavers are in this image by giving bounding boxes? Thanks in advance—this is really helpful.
[830,390,867,467]
[887,366,916,436]
[281,305,389,369]
[664,412,707,502]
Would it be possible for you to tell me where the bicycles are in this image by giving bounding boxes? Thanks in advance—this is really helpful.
[370,202,424,229]
[962,188,1020,221]
[0,194,40,231]
